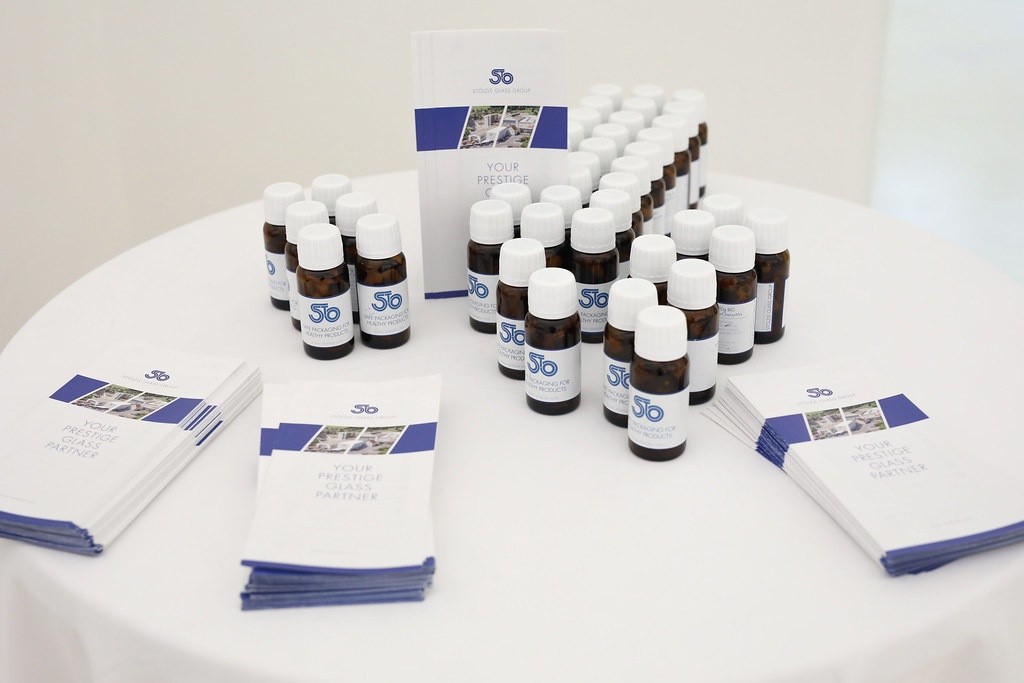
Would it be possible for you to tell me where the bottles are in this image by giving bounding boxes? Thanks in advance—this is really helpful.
[356,213,410,349]
[496,238,546,380]
[667,258,719,405]
[540,83,715,344]
[750,216,791,344]
[285,200,330,332]
[710,225,757,365]
[524,267,581,415]
[297,224,354,361]
[699,196,746,227]
[336,192,378,325]
[521,203,567,267]
[628,305,689,462]
[468,200,514,334]
[604,278,659,428]
[313,174,351,224]
[490,182,533,239]
[263,182,305,311]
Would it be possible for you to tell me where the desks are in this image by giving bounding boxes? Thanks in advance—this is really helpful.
[0,171,1024,683]
